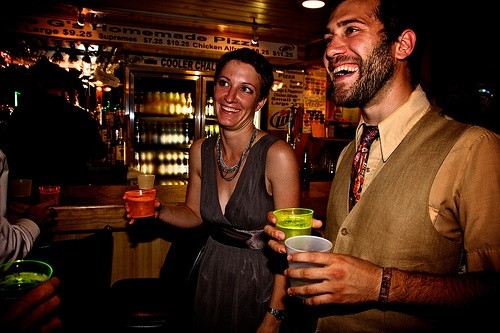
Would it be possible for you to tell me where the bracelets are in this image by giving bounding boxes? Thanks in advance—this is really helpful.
[378,266,392,304]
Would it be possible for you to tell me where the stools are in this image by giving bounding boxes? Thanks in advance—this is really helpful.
[110,237,207,333]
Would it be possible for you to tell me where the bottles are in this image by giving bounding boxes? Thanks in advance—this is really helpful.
[299,148,312,191]
[63,88,126,167]
[131,91,221,177]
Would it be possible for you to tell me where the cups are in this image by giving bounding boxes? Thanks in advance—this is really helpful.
[284,235,334,299]
[11,179,33,198]
[274,208,315,247]
[0,260,53,309]
[38,185,60,205]
[125,189,157,219]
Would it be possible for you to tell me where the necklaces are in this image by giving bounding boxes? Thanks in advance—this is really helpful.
[216,128,258,181]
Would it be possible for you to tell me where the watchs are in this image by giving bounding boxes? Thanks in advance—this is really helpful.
[265,305,288,320]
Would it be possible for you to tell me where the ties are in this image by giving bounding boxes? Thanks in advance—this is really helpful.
[349,125,380,212]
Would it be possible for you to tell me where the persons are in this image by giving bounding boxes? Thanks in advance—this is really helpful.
[123,47,300,333]
[0,63,108,185]
[0,277,61,333]
[264,0,500,333]
[0,150,55,265]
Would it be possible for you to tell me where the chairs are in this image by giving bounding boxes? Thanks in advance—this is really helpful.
[23,225,114,333]
